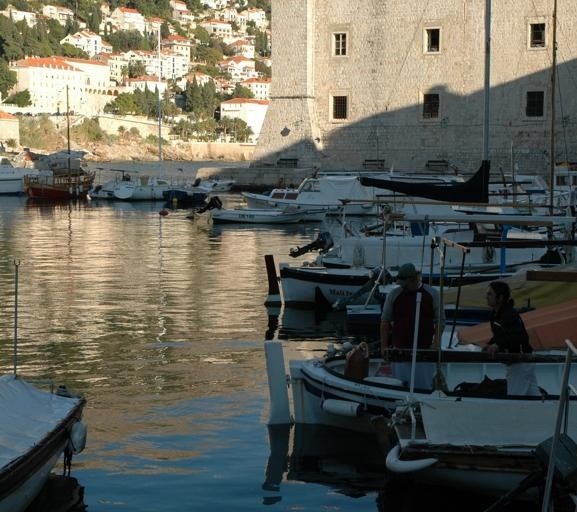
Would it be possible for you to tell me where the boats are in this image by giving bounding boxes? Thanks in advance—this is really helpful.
[0,373,86,512]
[0,86,237,200]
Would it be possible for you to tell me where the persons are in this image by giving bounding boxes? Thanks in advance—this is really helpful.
[379,260,446,390]
[480,279,541,397]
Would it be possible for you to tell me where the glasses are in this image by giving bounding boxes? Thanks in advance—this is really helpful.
[397,275,414,281]
